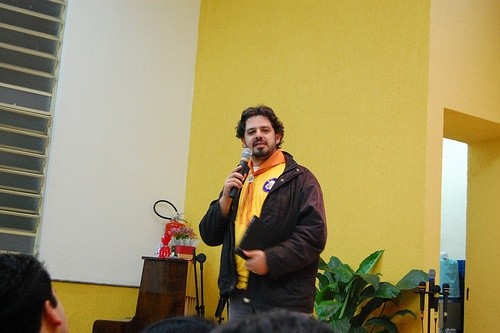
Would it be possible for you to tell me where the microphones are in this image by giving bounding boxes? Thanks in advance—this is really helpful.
[229,148,252,199]
[419,269,450,322]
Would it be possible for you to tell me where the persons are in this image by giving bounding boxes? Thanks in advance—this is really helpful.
[142,309,335,333]
[0,253,70,333]
[199,105,327,318]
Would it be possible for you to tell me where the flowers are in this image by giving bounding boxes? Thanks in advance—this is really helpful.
[167,224,194,241]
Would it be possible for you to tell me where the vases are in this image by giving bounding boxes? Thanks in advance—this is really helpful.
[174,241,197,260]
